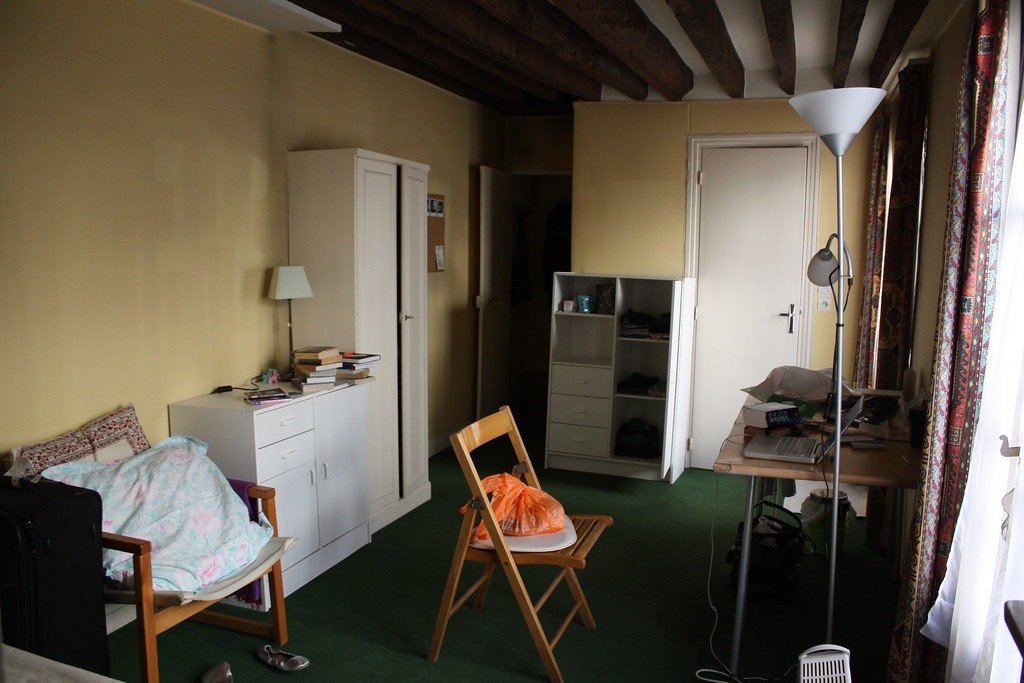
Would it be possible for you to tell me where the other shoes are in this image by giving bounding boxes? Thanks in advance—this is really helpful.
[258,645,310,672]
[202,661,234,683]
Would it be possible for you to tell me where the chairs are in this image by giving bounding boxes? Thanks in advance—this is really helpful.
[428,405,613,683]
[0,403,287,683]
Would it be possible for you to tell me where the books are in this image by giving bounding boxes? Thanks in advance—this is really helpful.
[244,388,292,404]
[843,423,911,449]
[291,346,381,393]
[743,402,800,437]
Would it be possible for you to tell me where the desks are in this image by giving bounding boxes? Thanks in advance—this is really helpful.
[713,389,921,680]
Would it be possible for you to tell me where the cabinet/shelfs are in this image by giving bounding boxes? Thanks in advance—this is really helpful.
[544,271,698,484]
[287,148,431,534]
[169,382,371,612]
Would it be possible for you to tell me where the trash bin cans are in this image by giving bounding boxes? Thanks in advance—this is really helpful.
[810,487,848,556]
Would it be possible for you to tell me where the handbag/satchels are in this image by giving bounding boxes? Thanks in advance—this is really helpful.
[614,417,657,459]
[726,500,802,612]
[460,473,566,538]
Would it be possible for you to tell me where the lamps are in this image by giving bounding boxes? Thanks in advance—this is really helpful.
[268,265,315,382]
[789,87,887,644]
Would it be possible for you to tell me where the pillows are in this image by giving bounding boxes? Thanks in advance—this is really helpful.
[41,435,275,595]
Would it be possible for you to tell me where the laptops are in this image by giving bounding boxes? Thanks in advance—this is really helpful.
[744,394,865,465]
